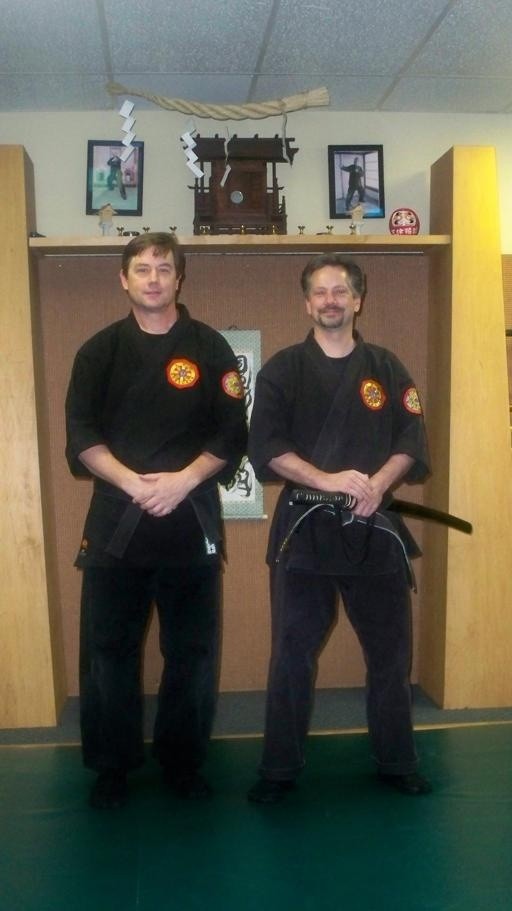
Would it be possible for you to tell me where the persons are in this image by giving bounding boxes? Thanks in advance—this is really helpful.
[107,152,127,199]
[245,252,433,804]
[338,157,367,209]
[65,232,250,810]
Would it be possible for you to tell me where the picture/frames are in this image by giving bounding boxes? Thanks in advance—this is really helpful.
[87,140,144,217]
[327,143,386,219]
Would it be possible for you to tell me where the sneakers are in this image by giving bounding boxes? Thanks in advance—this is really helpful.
[89,772,135,809]
[248,779,296,805]
[376,770,433,796]
[160,769,215,800]
